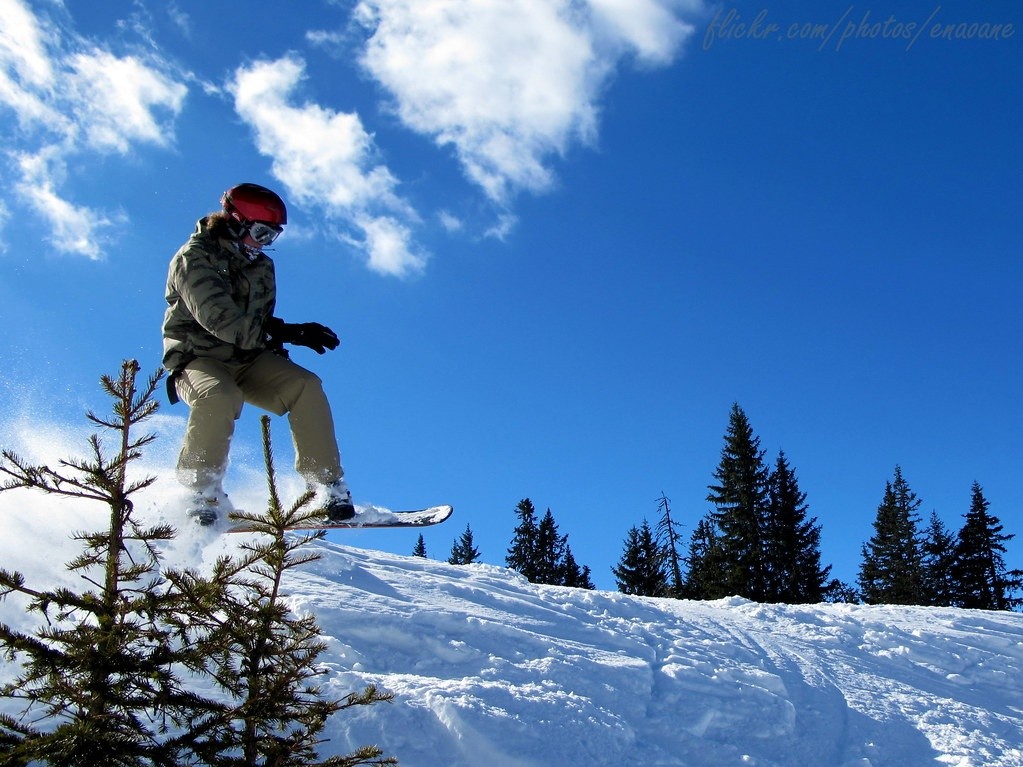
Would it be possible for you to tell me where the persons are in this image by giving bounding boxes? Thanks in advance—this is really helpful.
[161,183,355,525]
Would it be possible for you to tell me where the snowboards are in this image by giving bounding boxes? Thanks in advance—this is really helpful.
[230,503,456,534]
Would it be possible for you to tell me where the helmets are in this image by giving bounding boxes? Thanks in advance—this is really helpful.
[223,184,288,224]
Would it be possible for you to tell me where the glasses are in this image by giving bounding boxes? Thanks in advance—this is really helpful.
[249,225,283,246]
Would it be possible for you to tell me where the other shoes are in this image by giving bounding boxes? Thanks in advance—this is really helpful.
[325,481,354,520]
[187,493,217,526]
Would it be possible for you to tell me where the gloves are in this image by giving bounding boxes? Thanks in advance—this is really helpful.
[265,332,283,351]
[282,323,340,355]
